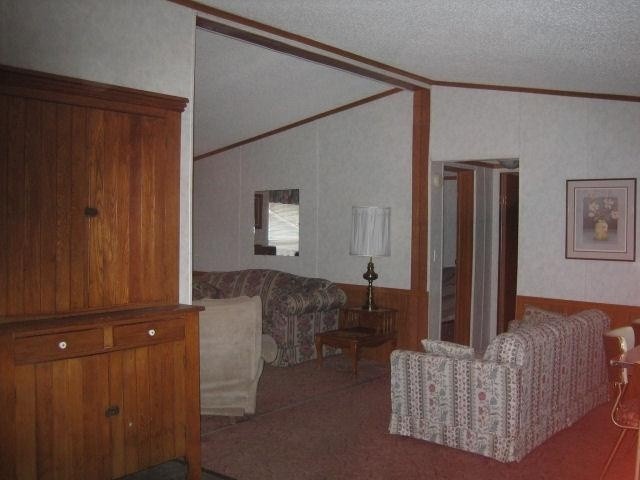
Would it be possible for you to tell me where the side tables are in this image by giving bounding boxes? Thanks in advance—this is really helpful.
[315,305,399,379]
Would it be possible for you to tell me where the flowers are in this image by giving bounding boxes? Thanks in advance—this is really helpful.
[585,198,621,219]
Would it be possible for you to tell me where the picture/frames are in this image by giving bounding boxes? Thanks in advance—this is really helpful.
[564,177,637,262]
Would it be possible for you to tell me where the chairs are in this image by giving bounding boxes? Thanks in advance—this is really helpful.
[193,296,263,425]
[598,325,640,479]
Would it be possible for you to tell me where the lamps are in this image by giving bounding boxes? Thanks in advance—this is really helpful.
[348,205,392,310]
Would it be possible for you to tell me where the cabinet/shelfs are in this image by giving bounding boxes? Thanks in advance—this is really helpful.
[1,65,190,317]
[0,303,203,480]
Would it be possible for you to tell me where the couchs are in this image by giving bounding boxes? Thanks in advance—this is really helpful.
[192,264,347,368]
[388,308,611,464]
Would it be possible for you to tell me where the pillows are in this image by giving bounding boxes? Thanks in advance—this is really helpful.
[420,338,476,359]
[519,305,562,330]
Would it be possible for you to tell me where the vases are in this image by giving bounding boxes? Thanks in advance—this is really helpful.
[594,219,609,241]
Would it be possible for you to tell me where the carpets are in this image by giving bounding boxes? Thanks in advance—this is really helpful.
[183,354,640,480]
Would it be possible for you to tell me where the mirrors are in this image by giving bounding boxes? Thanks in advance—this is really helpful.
[254,189,301,257]
